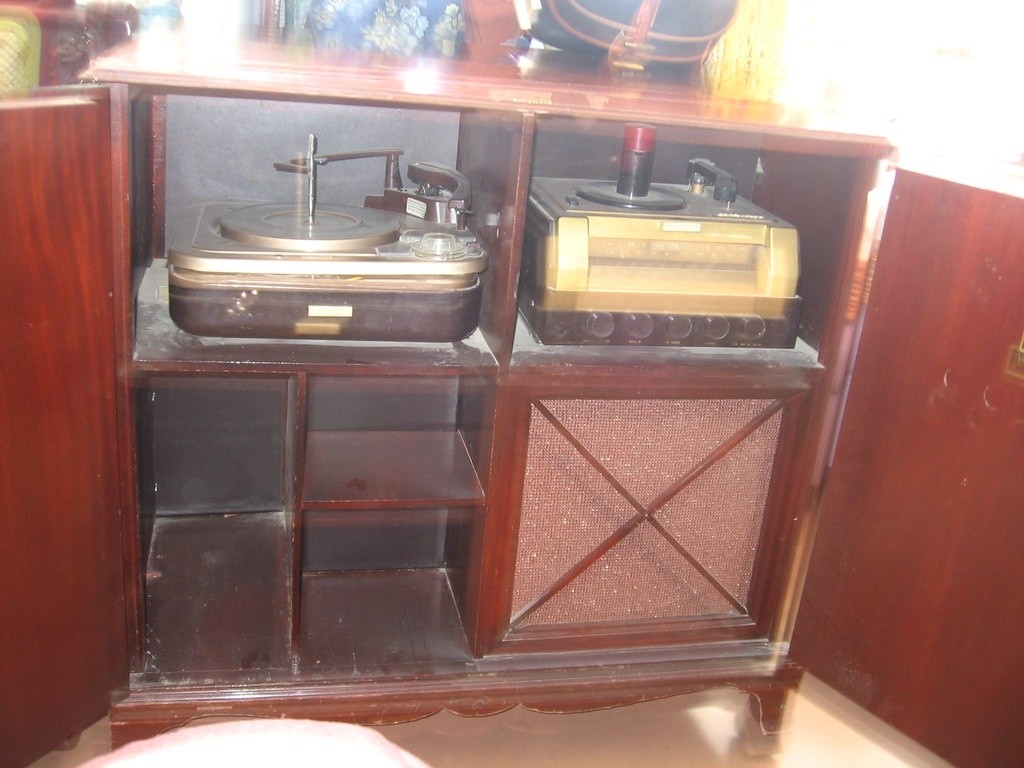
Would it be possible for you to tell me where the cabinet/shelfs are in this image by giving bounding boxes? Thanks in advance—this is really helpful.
[0,30,1024,768]
